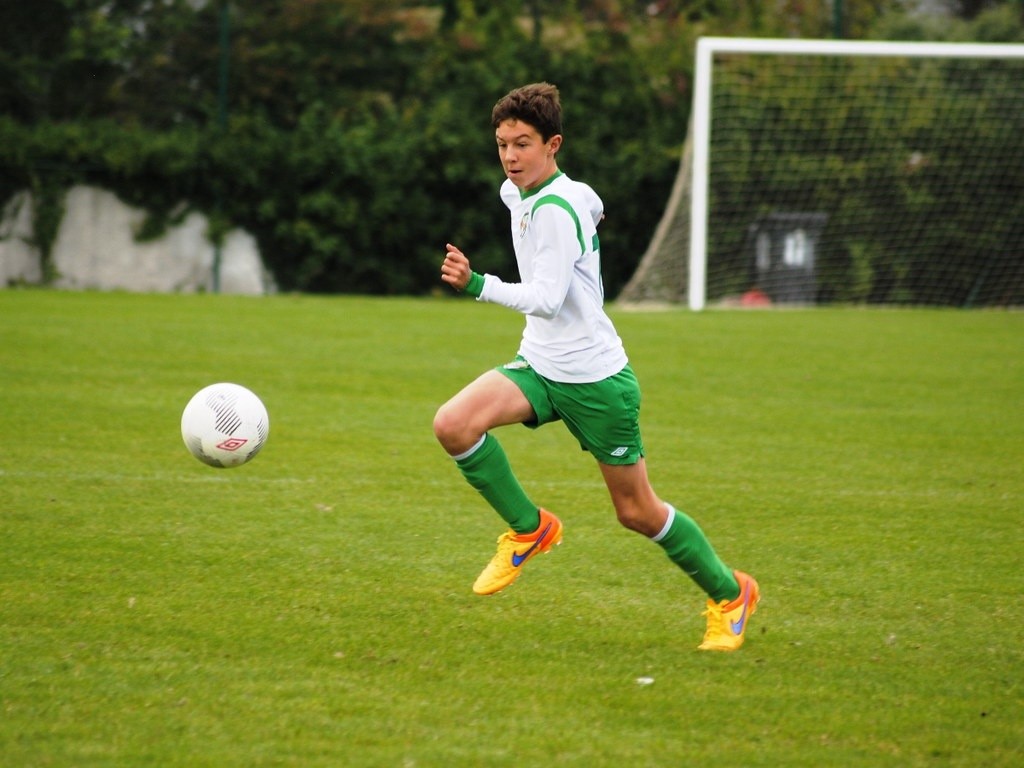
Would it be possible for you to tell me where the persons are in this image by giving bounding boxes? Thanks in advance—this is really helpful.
[432,82,763,650]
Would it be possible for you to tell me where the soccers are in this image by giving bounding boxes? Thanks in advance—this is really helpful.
[179,381,270,468]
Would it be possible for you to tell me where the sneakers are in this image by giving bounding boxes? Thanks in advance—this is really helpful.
[470,508,563,596]
[697,568,761,653]
